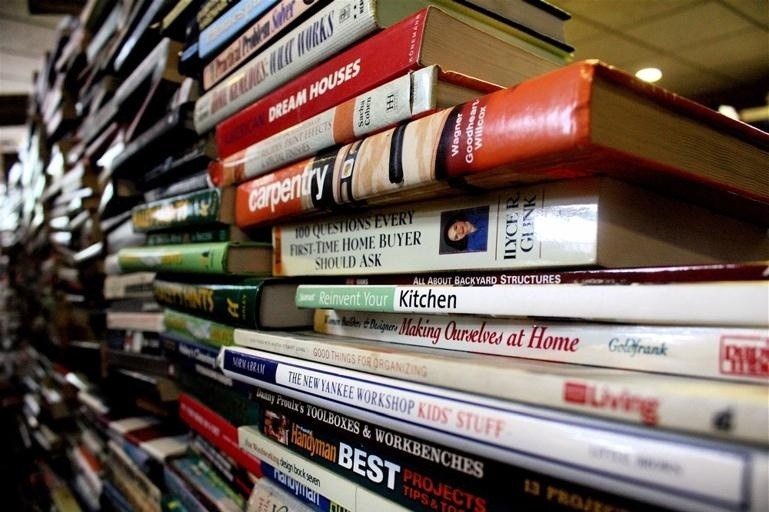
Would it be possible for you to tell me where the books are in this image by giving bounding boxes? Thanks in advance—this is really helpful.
[112,187,308,512]
[0,0,109,512]
[220,62,769,512]
[112,0,572,202]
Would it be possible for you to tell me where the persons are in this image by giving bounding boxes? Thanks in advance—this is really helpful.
[443,209,489,251]
